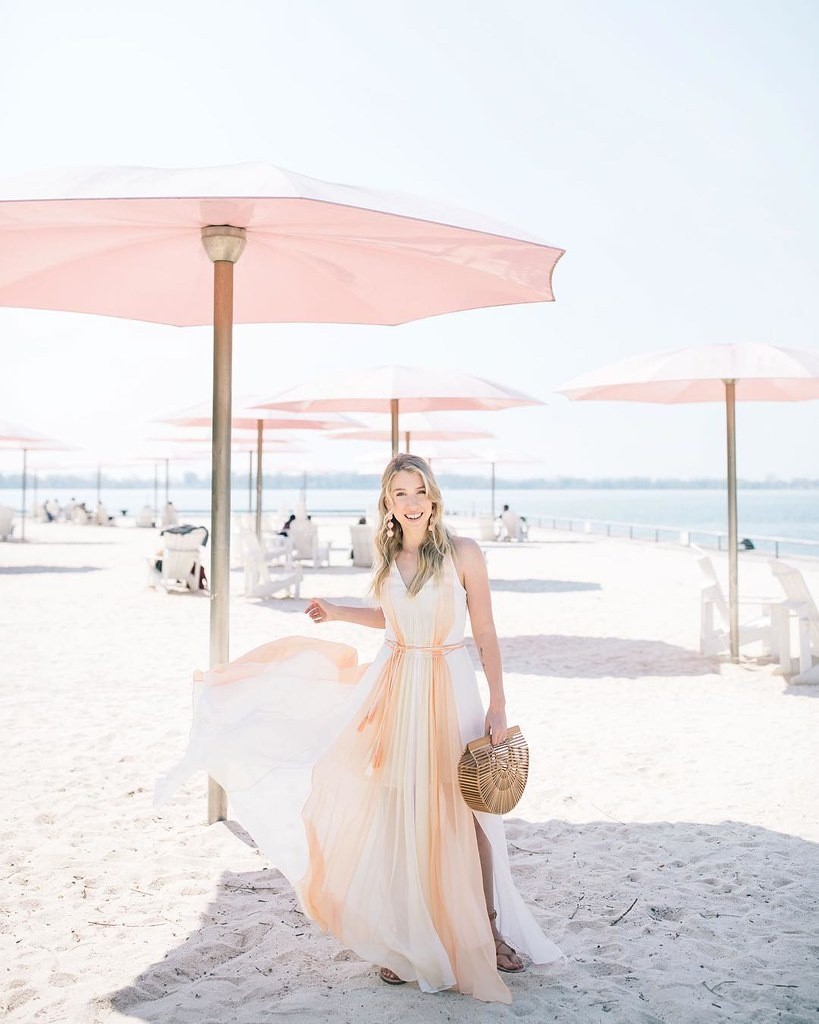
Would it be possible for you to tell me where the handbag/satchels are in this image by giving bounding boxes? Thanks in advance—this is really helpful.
[459,725,529,815]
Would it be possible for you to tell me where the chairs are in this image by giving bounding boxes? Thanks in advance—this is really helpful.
[768,559,819,685]
[236,531,302,601]
[0,506,16,541]
[128,525,210,595]
[288,504,330,570]
[696,556,777,659]
[349,524,375,567]
[30,500,177,529]
[501,511,522,541]
[479,515,497,540]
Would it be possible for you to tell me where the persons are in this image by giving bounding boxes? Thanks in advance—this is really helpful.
[278,515,295,537]
[494,505,510,541]
[304,453,530,985]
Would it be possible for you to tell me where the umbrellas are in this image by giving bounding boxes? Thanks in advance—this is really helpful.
[0,348,819,671]
[0,168,570,822]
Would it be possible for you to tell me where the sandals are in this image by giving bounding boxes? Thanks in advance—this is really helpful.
[379,967,407,985]
[488,910,526,973]
